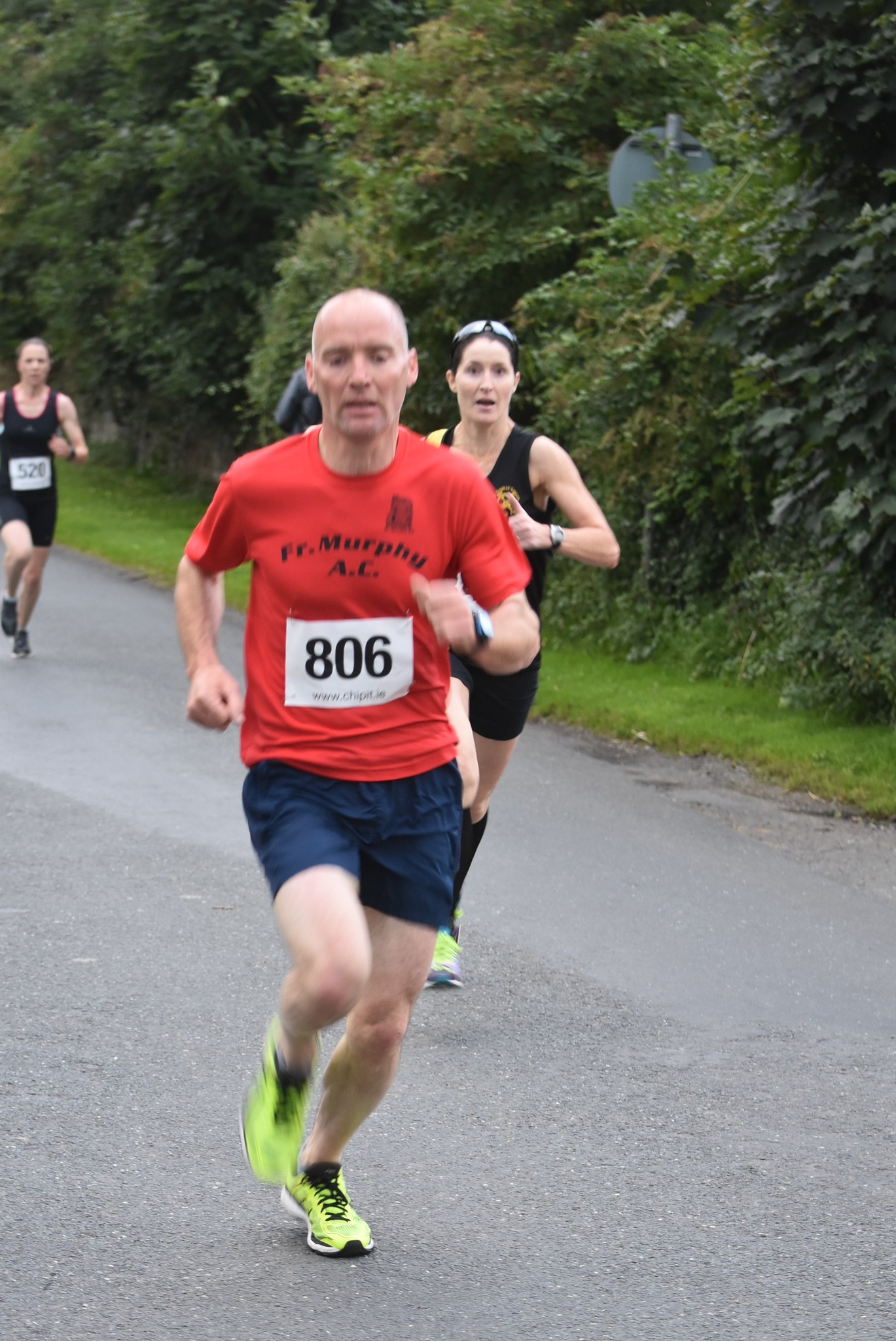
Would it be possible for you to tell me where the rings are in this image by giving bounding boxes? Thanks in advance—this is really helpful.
[516,535,521,539]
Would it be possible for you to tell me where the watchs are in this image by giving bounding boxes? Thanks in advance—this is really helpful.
[546,524,564,561]
[472,599,494,645]
[65,449,76,461]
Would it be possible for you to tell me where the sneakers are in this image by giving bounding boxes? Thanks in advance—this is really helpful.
[280,1163,374,1259]
[1,596,17,636]
[11,628,30,658]
[424,909,466,990]
[239,1016,323,1185]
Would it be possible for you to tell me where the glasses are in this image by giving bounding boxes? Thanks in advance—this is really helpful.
[452,319,517,349]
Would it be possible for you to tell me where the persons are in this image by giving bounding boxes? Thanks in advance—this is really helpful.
[273,368,322,434]
[0,336,89,658]
[420,320,621,987]
[173,288,541,1257]
